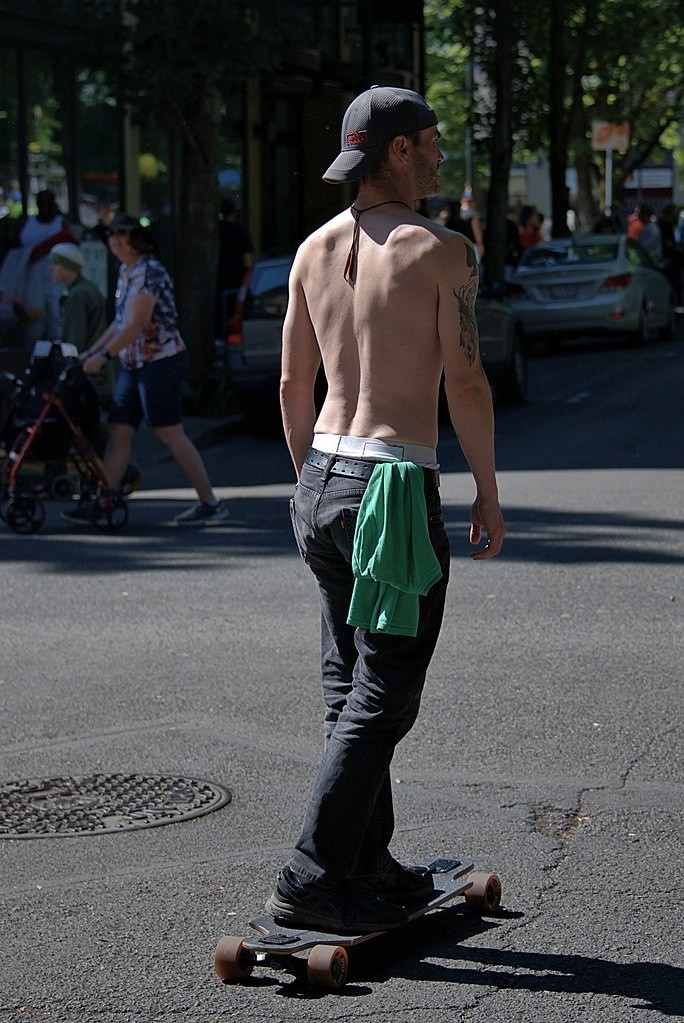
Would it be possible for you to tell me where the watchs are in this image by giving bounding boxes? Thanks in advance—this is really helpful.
[99,347,113,361]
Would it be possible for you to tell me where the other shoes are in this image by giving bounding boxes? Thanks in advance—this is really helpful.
[120,465,145,496]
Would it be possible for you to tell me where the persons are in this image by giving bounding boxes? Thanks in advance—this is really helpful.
[0,190,684,527]
[265,81,506,929]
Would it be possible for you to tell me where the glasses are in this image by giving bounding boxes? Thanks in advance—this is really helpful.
[106,228,128,238]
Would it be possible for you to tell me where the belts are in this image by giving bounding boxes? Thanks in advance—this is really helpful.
[305,449,440,488]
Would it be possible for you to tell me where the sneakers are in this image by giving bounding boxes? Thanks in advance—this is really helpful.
[60,503,116,525]
[266,868,408,931]
[175,500,230,525]
[350,848,435,901]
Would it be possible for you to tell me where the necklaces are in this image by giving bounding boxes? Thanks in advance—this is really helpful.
[341,201,414,282]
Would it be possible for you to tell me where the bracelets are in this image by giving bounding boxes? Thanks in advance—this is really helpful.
[84,348,93,355]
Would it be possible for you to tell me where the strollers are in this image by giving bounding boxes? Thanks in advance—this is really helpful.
[0,353,130,536]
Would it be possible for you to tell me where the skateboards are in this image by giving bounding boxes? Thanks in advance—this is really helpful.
[214,857,502,990]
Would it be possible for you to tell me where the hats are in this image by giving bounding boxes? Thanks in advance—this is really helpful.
[321,84,438,184]
[50,245,85,273]
[108,215,143,230]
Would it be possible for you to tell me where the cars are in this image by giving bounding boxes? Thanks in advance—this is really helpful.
[220,255,529,409]
[501,234,676,355]
